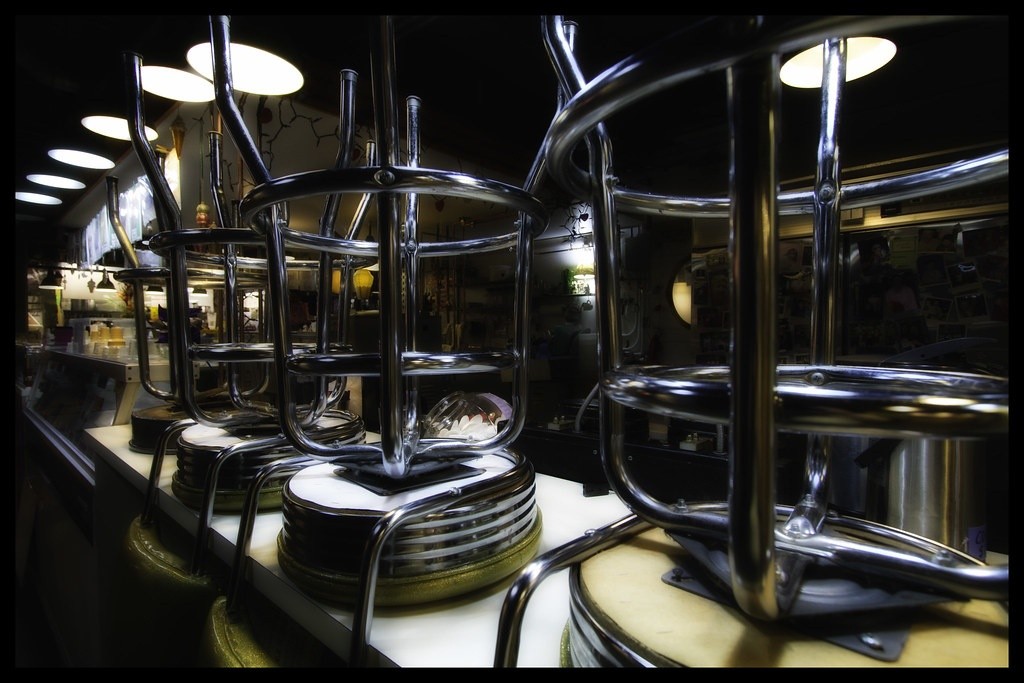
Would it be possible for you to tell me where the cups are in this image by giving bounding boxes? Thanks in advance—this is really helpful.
[101,327,110,342]
[111,327,123,339]
[92,330,98,341]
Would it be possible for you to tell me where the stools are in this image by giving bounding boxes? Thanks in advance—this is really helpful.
[104,13,1009,669]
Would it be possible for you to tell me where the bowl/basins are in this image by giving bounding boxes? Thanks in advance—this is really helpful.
[71,299,95,311]
